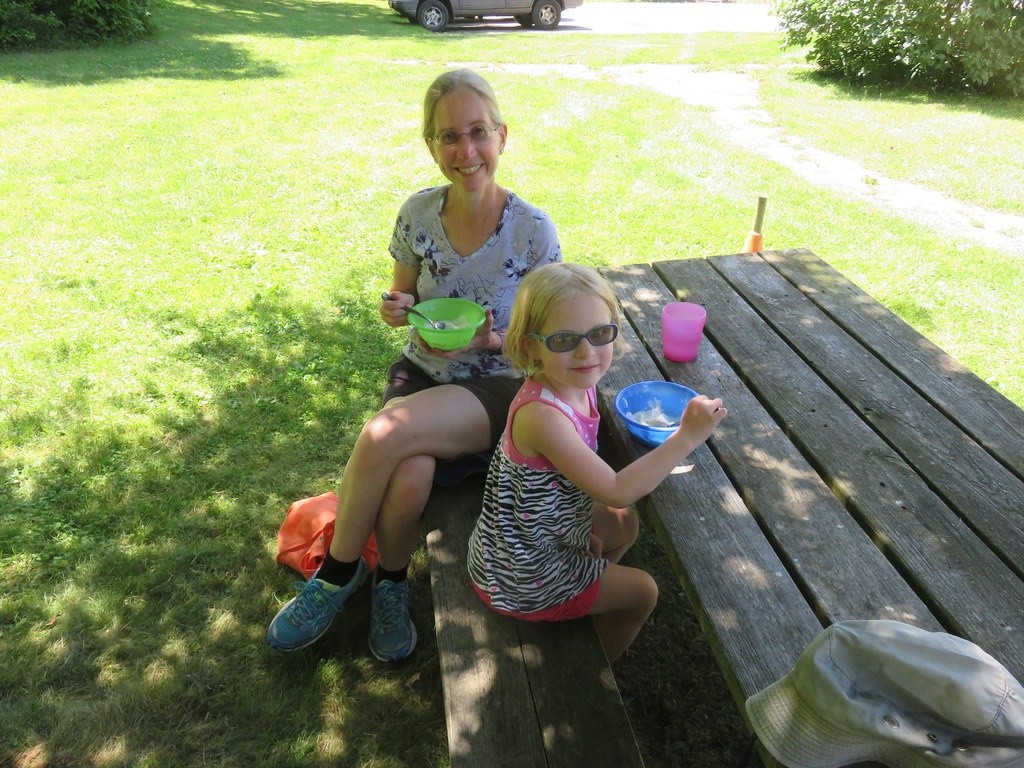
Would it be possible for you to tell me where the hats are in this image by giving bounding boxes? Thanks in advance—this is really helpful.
[744,619,1024,767]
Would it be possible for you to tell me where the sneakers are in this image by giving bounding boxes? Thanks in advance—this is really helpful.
[369,574,416,663]
[267,555,367,653]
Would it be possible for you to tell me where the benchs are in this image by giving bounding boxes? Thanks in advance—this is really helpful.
[418,447,646,768]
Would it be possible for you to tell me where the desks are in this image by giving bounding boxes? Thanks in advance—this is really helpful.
[592,248,1024,768]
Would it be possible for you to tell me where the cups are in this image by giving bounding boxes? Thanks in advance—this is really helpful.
[661,302,706,363]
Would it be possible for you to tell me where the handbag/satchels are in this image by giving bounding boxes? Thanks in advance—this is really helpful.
[275,491,382,581]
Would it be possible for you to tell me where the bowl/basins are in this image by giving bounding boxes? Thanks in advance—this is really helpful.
[613,380,701,446]
[407,297,487,351]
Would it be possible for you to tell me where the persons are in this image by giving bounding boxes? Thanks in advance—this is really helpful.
[266,65,563,660]
[463,261,728,665]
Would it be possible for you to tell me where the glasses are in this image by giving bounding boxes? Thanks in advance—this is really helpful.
[532,322,619,352]
[429,124,502,149]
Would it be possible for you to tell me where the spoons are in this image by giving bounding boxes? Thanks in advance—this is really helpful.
[382,292,457,331]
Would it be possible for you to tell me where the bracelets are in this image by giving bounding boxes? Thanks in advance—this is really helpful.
[489,329,507,355]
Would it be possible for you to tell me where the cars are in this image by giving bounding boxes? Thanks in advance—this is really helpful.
[389,0,585,33]
